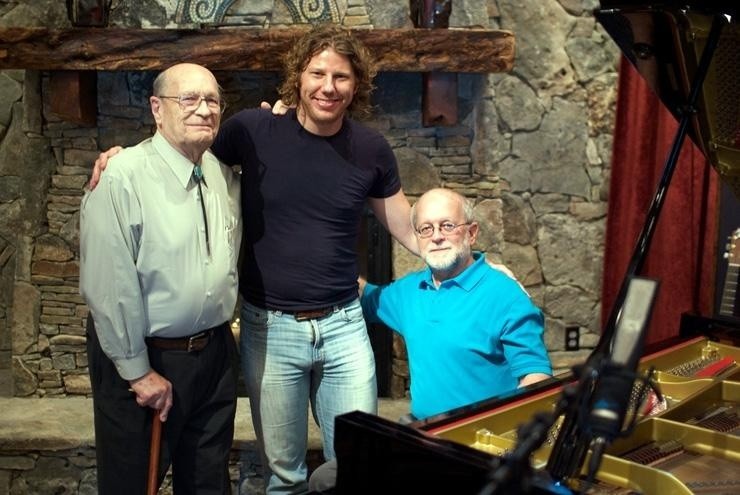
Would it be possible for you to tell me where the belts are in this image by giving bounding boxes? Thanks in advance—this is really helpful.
[145,329,214,352]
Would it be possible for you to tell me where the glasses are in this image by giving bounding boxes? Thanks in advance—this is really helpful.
[418,221,465,237]
[159,95,226,112]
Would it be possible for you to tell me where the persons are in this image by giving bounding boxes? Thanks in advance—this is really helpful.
[308,187,555,495]
[76,60,300,495]
[89,20,531,495]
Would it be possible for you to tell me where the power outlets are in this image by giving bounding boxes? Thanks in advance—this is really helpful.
[565,326,579,351]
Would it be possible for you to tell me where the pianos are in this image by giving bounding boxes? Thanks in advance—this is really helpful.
[334,3,740,495]
